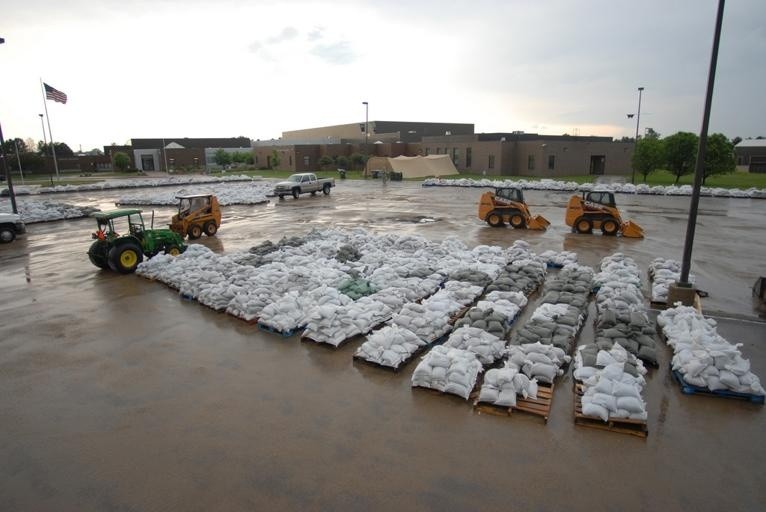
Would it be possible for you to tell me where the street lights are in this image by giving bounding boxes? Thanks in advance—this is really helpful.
[362,102,369,178]
[39,113,54,184]
[633,87,644,182]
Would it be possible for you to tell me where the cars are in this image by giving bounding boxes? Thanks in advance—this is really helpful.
[0,213,25,243]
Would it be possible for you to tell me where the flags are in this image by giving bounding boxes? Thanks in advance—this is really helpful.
[43,82,68,104]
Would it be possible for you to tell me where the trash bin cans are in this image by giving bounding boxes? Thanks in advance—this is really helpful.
[390,171,403,181]
[339,171,346,178]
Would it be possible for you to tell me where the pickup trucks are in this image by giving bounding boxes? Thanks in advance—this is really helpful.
[273,173,336,199]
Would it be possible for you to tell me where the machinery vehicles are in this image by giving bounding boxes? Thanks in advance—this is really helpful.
[479,185,644,239]
[86,194,221,275]
